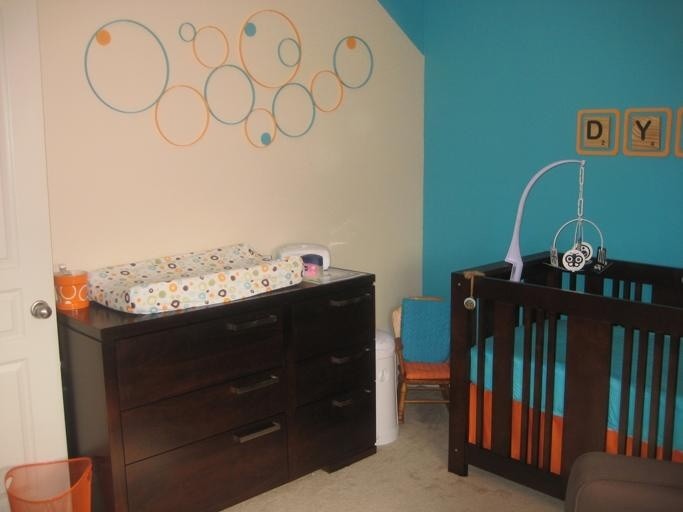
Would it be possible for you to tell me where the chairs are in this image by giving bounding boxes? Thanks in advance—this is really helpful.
[392,295,450,424]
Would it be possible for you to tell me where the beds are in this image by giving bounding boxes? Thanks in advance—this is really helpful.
[447,250,682,504]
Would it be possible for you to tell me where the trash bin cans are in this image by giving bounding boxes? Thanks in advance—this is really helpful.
[374,327,399,446]
[4,457,93,512]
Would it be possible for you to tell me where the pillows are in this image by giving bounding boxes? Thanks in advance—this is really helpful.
[399,299,450,364]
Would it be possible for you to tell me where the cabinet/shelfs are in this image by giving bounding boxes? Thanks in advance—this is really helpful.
[57,265,377,511]
